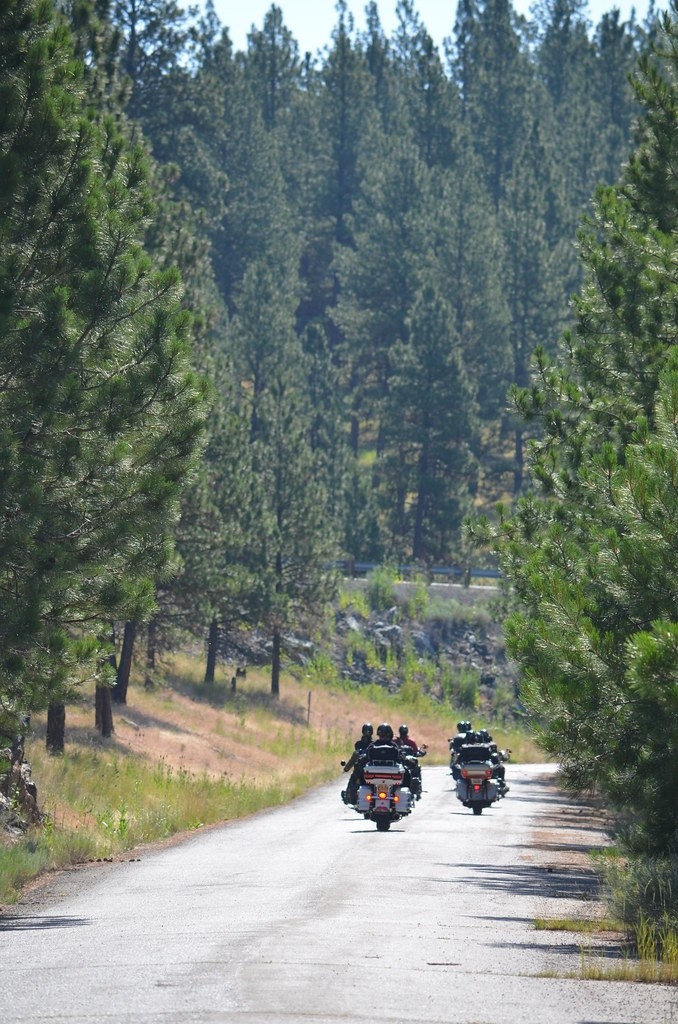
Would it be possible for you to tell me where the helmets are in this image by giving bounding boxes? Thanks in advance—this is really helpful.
[466,729,489,743]
[377,724,393,738]
[457,720,471,732]
[399,725,408,734]
[362,724,373,735]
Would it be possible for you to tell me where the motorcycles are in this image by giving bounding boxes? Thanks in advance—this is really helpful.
[339,743,428,819]
[448,731,513,815]
[350,744,415,832]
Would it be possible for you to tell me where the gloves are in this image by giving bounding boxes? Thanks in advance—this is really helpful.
[418,749,426,756]
[406,758,415,767]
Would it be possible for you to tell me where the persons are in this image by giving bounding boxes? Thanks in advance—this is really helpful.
[450,722,511,794]
[353,724,412,790]
[392,726,418,755]
[354,725,375,753]
